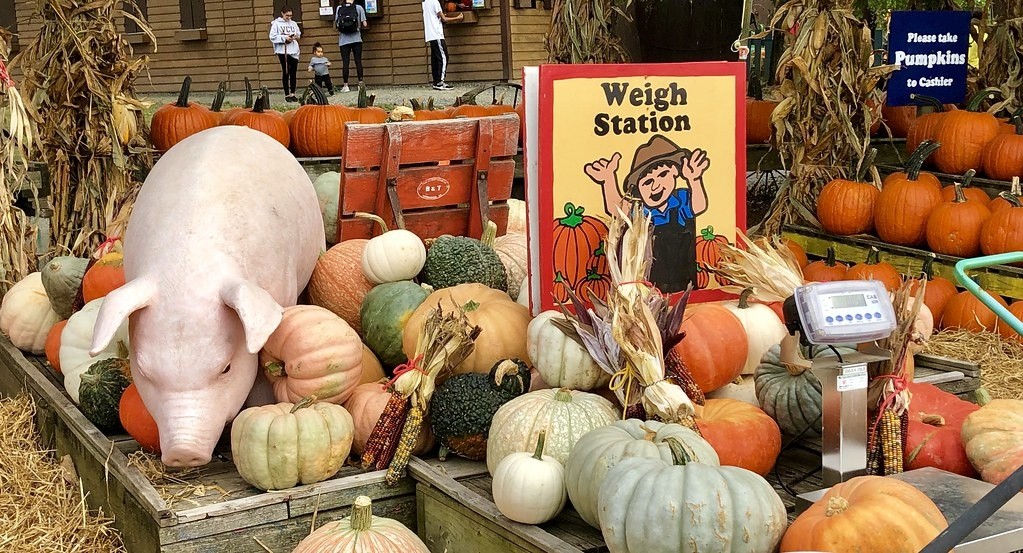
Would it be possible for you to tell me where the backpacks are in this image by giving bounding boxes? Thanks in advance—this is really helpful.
[336,3,358,34]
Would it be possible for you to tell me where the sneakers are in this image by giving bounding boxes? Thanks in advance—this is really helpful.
[433,81,454,90]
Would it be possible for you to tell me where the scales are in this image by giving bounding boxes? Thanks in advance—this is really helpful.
[782,280,1023,553]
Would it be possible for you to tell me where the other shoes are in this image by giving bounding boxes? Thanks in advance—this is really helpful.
[292,96,297,102]
[285,96,293,102]
[340,86,350,93]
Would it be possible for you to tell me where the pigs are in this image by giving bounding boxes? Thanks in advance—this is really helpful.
[87,122,327,470]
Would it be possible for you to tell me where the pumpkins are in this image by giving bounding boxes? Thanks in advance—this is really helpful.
[0,74,1023,553]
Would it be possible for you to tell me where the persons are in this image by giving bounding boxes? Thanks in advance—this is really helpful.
[332,0,367,92]
[269,6,300,103]
[423,0,463,90]
[307,42,334,96]
